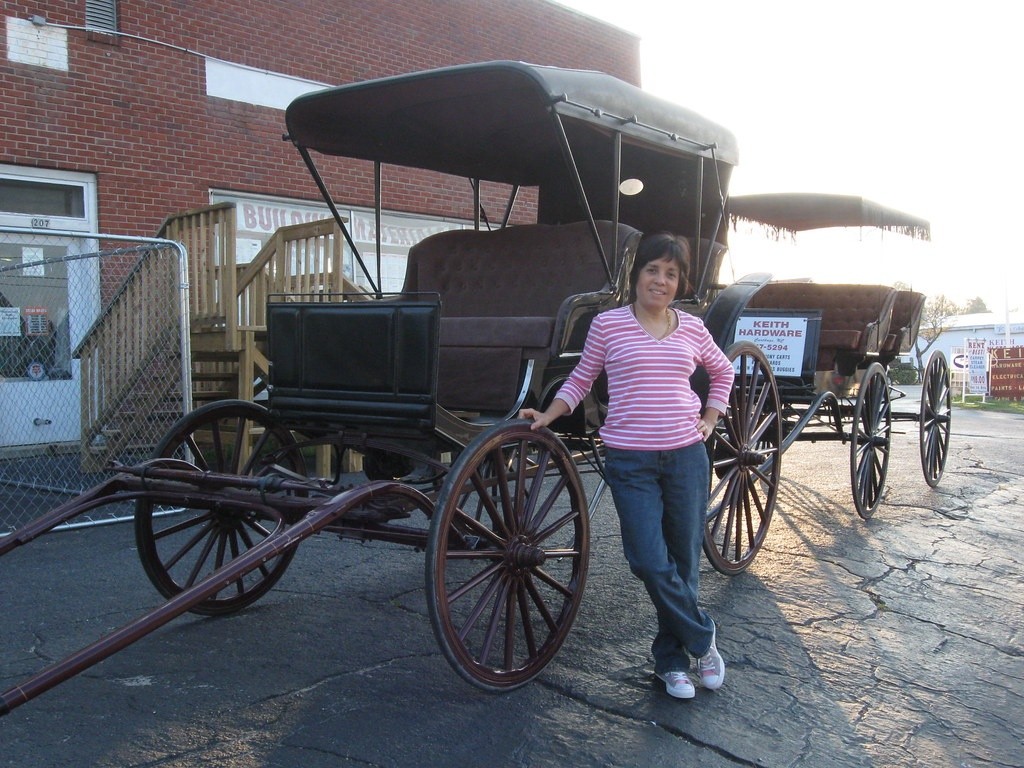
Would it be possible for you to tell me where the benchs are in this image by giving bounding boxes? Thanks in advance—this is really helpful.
[880,291,926,353]
[395,219,642,411]
[744,282,899,371]
[627,236,729,317]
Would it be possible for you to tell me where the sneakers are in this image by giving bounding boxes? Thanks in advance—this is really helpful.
[653,662,695,699]
[696,619,726,690]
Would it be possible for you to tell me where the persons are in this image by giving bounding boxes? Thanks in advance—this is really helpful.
[517,234,735,697]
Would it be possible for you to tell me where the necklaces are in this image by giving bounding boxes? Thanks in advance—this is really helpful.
[633,302,671,337]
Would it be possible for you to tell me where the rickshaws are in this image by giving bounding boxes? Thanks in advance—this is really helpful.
[704,192,951,522]
[0,59,782,717]
